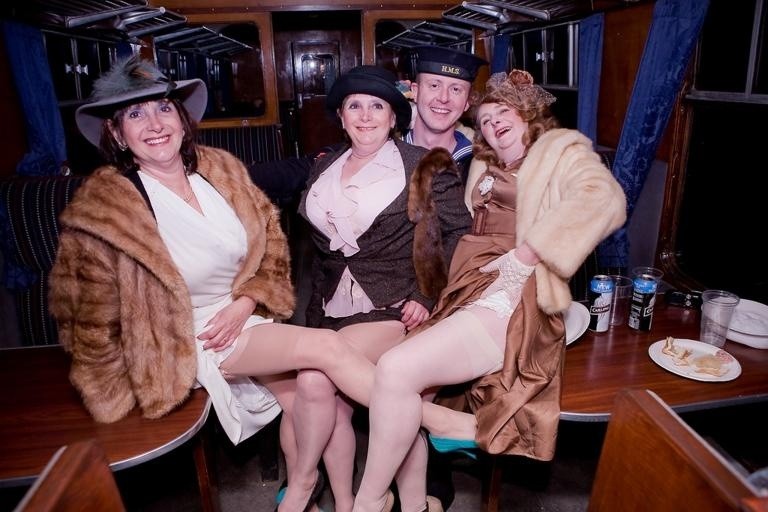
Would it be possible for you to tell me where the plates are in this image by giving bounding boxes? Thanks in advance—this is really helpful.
[702,297,768,337]
[706,319,768,350]
[566,300,590,346]
[650,337,742,382]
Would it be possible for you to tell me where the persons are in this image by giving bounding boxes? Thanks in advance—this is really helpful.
[349,70,629,511]
[46,54,484,512]
[271,64,474,511]
[395,42,489,188]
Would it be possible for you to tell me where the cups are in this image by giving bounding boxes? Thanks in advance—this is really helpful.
[608,273,634,328]
[632,266,663,284]
[699,290,739,345]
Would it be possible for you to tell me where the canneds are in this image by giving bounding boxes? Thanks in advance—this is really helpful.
[587,274,613,333]
[627,274,657,332]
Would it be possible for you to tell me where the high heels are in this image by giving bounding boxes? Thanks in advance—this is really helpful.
[429,436,481,460]
[275,471,324,512]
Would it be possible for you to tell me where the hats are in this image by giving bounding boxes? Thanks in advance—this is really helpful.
[326,65,413,130]
[75,63,208,150]
[412,44,488,79]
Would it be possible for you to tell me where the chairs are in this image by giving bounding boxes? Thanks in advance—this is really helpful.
[2,176,82,348]
[0,433,122,512]
[194,124,287,184]
[589,385,767,510]
